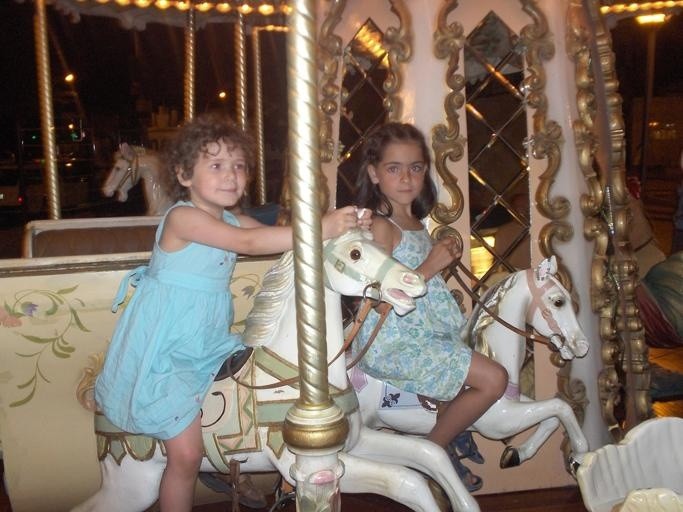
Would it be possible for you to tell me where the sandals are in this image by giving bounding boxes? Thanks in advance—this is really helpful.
[199,473,267,508]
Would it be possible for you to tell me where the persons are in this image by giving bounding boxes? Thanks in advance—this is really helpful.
[351,123,506,492]
[95,112,373,511]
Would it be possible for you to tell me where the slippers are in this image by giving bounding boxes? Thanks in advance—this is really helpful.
[456,433,484,463]
[445,447,483,491]
